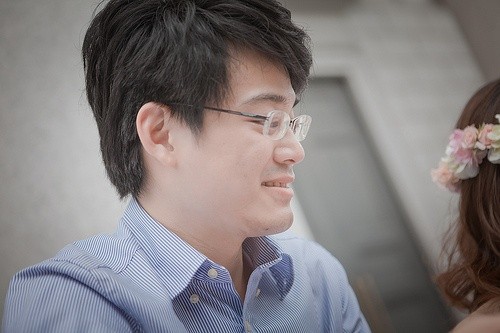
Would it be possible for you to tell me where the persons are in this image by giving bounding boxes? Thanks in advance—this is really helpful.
[429,79,500,332]
[0,0,372,333]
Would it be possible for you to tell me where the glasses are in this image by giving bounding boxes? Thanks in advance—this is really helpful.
[165,102,312,141]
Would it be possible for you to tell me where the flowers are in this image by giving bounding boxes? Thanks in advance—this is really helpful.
[430,113,500,192]
